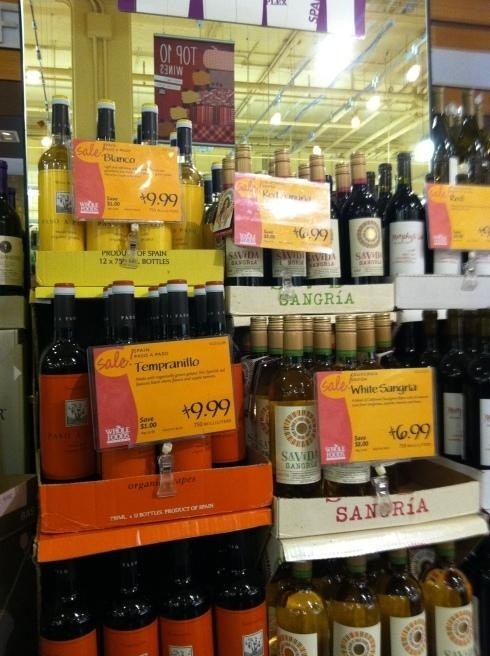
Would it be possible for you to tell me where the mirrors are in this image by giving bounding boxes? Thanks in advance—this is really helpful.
[19,0,434,251]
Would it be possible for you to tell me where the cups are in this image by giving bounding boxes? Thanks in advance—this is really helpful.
[39,524,267,655]
[223,93,489,285]
[268,540,488,656]
[44,280,250,484]
[249,314,413,498]
[42,98,225,250]
[394,312,490,469]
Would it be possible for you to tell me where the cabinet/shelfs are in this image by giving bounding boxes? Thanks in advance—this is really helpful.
[34,183,490,656]
[0,155,33,655]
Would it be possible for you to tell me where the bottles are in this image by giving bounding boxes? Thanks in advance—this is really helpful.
[0,159,29,295]
